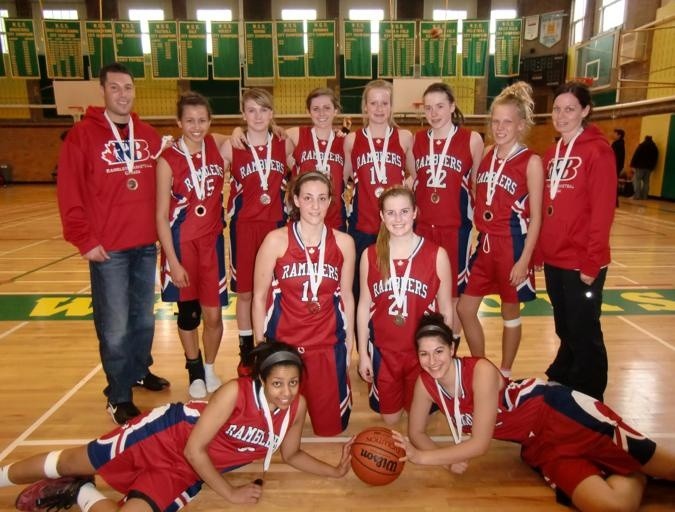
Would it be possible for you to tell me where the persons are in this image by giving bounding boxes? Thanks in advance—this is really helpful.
[629,137,658,200]
[156,79,545,436]
[392,312,674,512]
[611,129,625,207]
[534,82,619,403]
[58,63,161,427]
[3,341,356,512]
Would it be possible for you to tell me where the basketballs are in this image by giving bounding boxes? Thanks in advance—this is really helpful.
[350,427,405,484]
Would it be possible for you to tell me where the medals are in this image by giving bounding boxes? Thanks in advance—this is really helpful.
[547,200,554,216]
[127,173,138,191]
[253,478,263,486]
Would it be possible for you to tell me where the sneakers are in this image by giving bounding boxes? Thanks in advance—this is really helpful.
[15,475,94,512]
[189,364,222,398]
[106,398,141,424]
[132,372,170,391]
